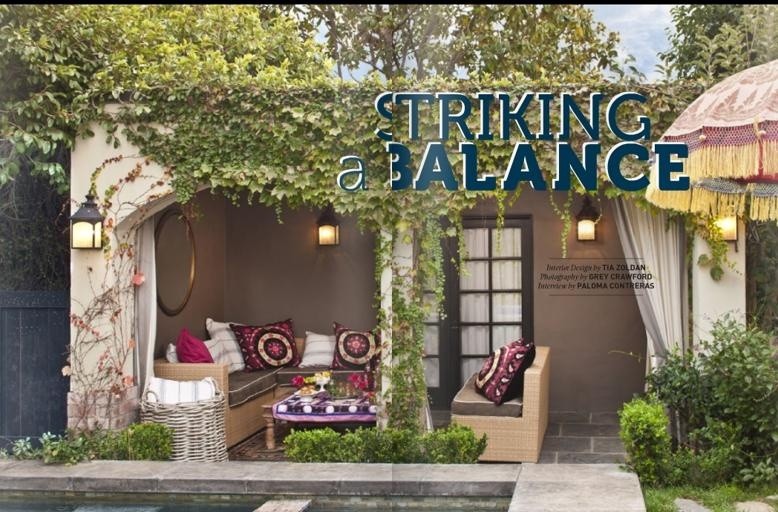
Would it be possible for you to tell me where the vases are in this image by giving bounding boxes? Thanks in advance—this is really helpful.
[315,379,329,392]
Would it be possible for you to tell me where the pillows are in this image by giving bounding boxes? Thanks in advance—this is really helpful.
[475,338,536,406]
[165,318,380,374]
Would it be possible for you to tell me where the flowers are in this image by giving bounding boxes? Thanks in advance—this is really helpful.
[291,370,333,389]
[347,372,370,390]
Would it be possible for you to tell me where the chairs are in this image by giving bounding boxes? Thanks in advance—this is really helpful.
[450,345,552,464]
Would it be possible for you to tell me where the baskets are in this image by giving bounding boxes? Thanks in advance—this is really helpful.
[137,376,229,463]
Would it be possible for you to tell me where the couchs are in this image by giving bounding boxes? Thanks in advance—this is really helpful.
[153,336,381,451]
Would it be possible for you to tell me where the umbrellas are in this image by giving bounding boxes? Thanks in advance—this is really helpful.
[645,58,778,220]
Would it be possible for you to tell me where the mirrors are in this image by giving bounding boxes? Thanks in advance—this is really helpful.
[154,207,196,316]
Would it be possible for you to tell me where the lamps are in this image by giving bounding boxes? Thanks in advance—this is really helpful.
[316,204,340,246]
[68,191,105,250]
[573,195,599,242]
[710,213,739,252]
